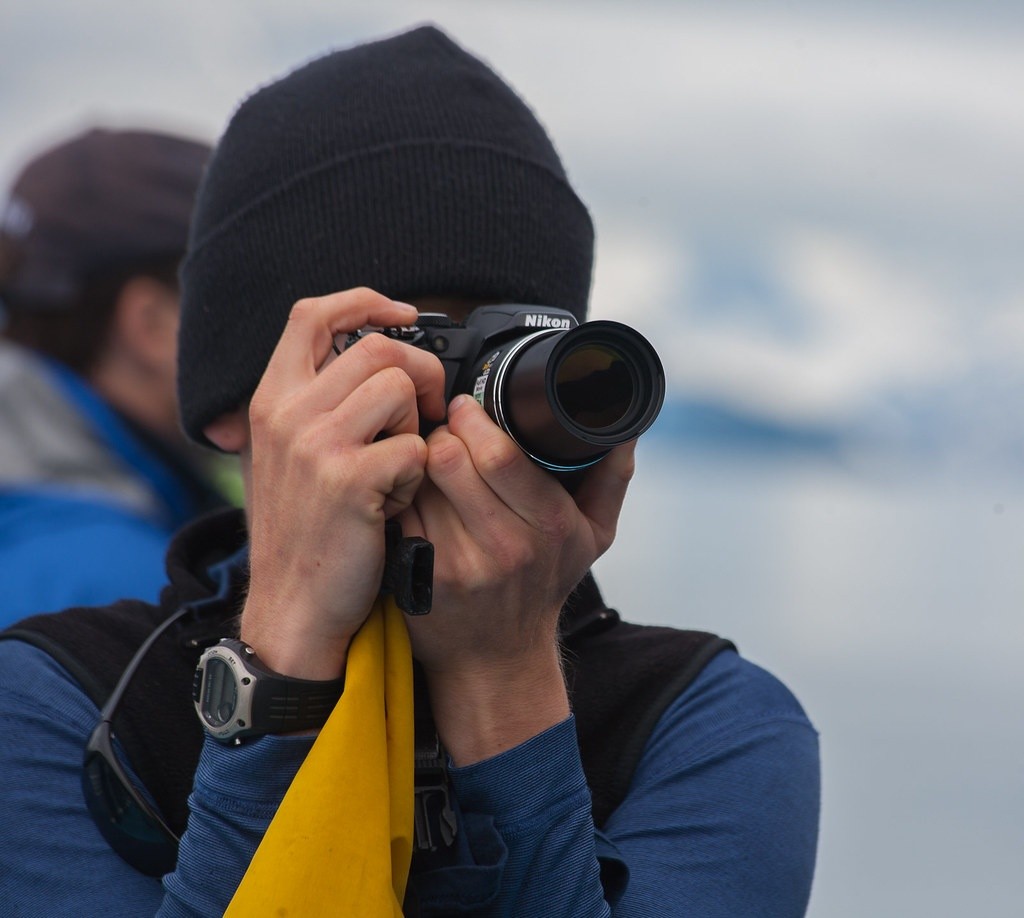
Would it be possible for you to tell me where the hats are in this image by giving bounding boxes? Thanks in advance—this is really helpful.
[0,124,214,368]
[175,25,593,454]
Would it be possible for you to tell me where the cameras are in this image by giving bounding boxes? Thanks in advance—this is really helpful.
[345,303,665,493]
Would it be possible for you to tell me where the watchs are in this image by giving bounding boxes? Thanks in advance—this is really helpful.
[191,638,346,745]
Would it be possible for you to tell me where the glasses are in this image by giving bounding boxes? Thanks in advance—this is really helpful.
[80,606,183,879]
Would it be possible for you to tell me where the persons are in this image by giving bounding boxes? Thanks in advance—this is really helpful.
[0,124,252,626]
[0,26,821,918]
